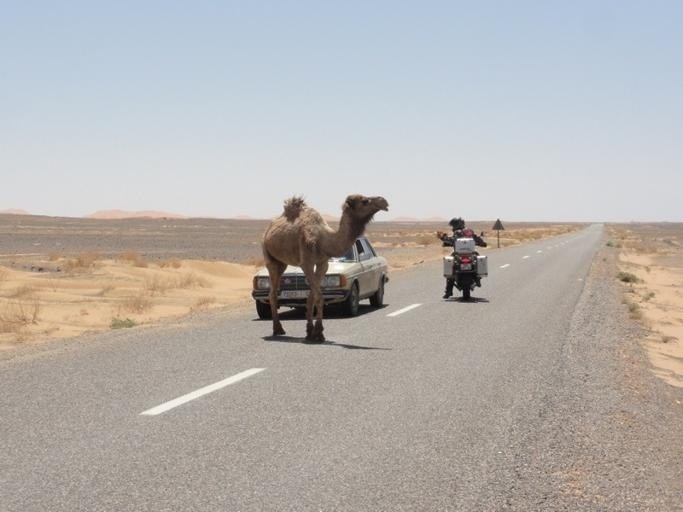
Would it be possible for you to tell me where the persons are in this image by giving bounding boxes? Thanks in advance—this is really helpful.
[437,217,487,299]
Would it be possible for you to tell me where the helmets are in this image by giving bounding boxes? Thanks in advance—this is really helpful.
[448,218,465,231]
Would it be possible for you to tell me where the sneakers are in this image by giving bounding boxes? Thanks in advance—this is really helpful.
[444,290,453,299]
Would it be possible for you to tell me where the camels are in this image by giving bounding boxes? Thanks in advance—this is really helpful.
[261,195,388,342]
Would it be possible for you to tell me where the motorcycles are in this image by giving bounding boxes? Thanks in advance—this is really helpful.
[443,231,489,300]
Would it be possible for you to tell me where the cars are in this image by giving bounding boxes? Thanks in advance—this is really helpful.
[252,235,389,320]
